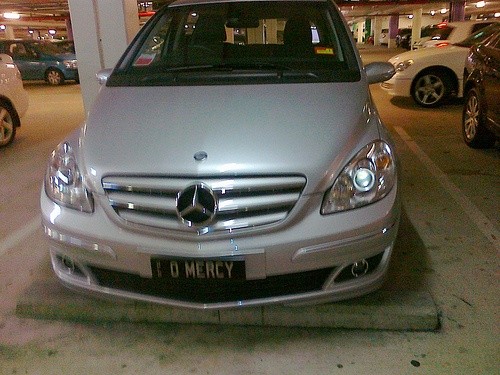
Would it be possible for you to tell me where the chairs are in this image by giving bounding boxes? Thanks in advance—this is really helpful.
[184,14,236,59]
[274,17,315,58]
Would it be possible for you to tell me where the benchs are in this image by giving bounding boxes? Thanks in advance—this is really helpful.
[237,44,279,58]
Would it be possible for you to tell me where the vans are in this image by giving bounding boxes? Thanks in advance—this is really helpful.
[379,21,491,51]
[0,39,77,87]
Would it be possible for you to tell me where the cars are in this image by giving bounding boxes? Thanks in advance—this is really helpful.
[382,23,500,148]
[0,53,29,155]
[41,0,402,311]
